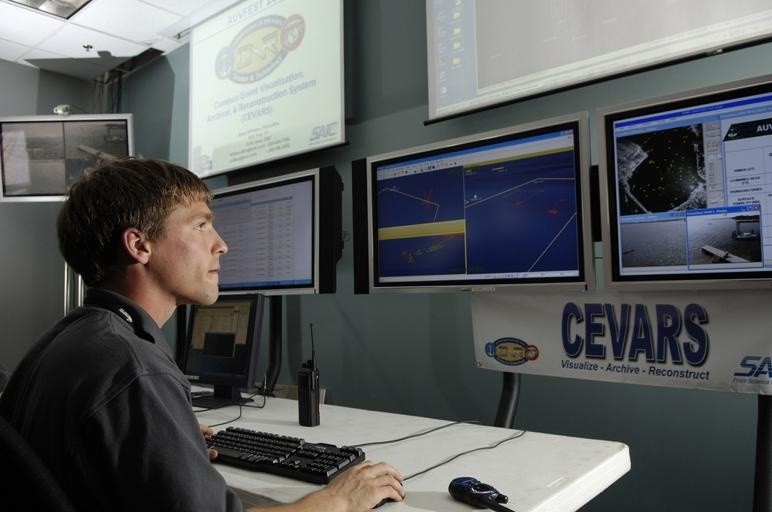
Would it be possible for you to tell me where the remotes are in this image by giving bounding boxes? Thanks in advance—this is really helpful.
[372,497,393,509]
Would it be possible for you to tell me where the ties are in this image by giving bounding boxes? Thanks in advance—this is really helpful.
[365,110,596,294]
[596,74,772,292]
[206,167,320,296]
[0,113,135,202]
[179,294,265,409]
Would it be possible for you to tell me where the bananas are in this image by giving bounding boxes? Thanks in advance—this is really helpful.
[205,427,365,485]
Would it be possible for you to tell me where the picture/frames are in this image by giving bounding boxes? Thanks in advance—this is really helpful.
[186,386,633,512]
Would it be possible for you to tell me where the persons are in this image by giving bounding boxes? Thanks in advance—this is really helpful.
[0,155,408,512]
